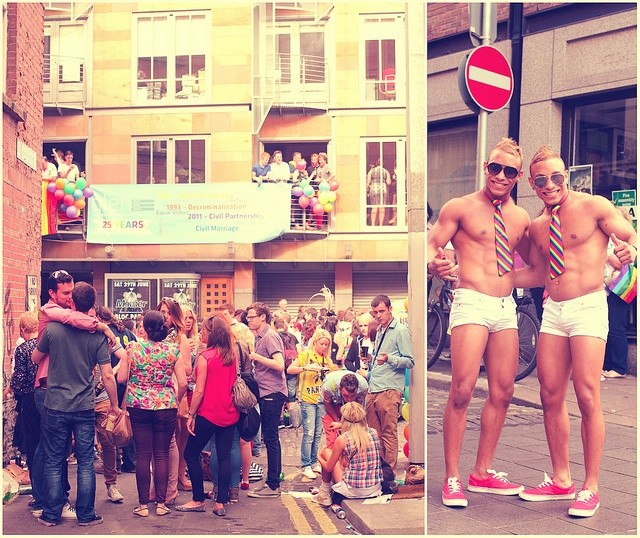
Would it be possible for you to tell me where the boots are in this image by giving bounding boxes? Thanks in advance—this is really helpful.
[211,486,218,501]
[229,487,239,504]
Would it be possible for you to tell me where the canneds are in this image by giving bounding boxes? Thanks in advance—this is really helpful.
[17,485,34,495]
[265,472,284,481]
[336,508,347,520]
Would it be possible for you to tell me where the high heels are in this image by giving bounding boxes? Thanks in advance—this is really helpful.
[156,505,171,515]
[133,507,149,517]
[154,492,181,504]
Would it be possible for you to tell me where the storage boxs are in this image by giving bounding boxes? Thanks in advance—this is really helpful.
[181,74,197,88]
[198,69,207,95]
[146,81,155,100]
[153,81,161,100]
[182,87,192,95]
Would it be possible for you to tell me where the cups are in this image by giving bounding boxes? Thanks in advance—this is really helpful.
[361,346,369,355]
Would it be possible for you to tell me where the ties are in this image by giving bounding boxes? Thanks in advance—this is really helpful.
[543,192,569,280]
[483,188,514,277]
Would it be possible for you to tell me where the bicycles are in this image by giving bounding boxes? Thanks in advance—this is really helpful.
[428,274,541,381]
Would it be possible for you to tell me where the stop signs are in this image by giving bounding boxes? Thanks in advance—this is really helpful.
[465,46,515,111]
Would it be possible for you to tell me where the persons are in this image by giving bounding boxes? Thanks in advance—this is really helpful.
[93,305,138,475]
[239,437,252,490]
[357,295,415,468]
[120,318,135,334]
[291,305,376,350]
[241,311,257,338]
[269,150,290,183]
[294,159,308,182]
[636,329,637,332]
[180,304,198,413]
[118,310,188,517]
[514,146,635,517]
[67,431,103,462]
[270,313,296,334]
[287,329,336,479]
[602,206,632,378]
[219,304,256,376]
[427,202,436,305]
[272,299,289,318]
[289,152,301,223]
[247,302,289,498]
[3,312,48,506]
[324,317,344,368]
[427,137,533,508]
[311,401,384,506]
[174,315,239,516]
[317,370,369,452]
[158,296,194,491]
[251,402,262,457]
[12,336,24,376]
[41,156,58,181]
[389,161,398,225]
[344,314,374,381]
[51,148,65,166]
[195,313,250,503]
[307,153,320,227]
[196,318,203,339]
[274,319,300,429]
[234,308,245,322]
[439,241,459,360]
[398,315,409,328]
[335,319,361,371]
[252,152,272,185]
[139,318,147,341]
[57,150,80,182]
[359,321,379,382]
[367,158,391,226]
[314,153,335,182]
[85,303,124,502]
[571,169,591,193]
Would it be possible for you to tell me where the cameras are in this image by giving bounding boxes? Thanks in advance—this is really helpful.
[315,367,329,373]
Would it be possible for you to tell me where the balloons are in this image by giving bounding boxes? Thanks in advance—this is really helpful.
[75,209,80,218]
[405,368,409,387]
[75,178,86,190]
[299,195,309,209]
[73,190,83,200]
[404,425,409,441]
[319,192,329,204]
[82,188,94,198]
[303,185,314,196]
[47,182,57,193]
[318,191,321,196]
[313,204,324,215]
[329,180,339,191]
[74,200,85,209]
[323,203,333,213]
[82,196,85,200]
[61,204,67,212]
[293,187,303,197]
[54,189,64,200]
[69,183,76,190]
[404,297,409,310]
[63,195,74,206]
[296,159,307,171]
[404,442,409,458]
[308,190,314,197]
[63,179,69,184]
[56,178,64,189]
[328,191,336,202]
[66,205,77,218]
[404,386,410,403]
[300,179,308,190]
[310,198,318,207]
[85,181,90,188]
[63,184,73,194]
[319,182,330,192]
[401,403,410,423]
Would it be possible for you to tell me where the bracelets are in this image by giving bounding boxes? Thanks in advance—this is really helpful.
[188,411,195,415]
[631,245,637,251]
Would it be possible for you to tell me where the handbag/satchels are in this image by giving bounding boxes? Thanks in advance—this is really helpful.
[288,400,303,427]
[237,342,260,403]
[232,355,258,414]
[100,406,134,448]
[176,394,191,419]
[238,407,261,442]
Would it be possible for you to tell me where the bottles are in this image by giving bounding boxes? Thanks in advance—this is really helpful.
[265,473,284,480]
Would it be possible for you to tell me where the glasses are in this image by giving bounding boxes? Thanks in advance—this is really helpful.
[246,315,260,319]
[162,297,179,304]
[51,269,70,279]
[487,162,519,180]
[371,310,386,317]
[533,174,565,188]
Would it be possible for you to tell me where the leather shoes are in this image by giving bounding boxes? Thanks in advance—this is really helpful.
[604,370,627,378]
[212,507,226,516]
[175,504,207,512]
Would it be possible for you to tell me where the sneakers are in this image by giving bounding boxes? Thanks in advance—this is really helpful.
[246,484,279,498]
[106,485,124,502]
[28,499,37,505]
[568,484,600,517]
[468,469,525,496]
[78,515,103,526]
[178,478,193,490]
[300,467,317,479]
[312,464,321,472]
[441,477,468,508]
[36,515,57,527]
[310,493,332,506]
[31,509,42,517]
[252,484,281,496]
[94,463,121,475]
[240,482,249,490]
[519,472,576,501]
[60,502,77,520]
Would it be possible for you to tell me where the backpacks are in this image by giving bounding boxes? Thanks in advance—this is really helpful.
[279,331,300,369]
